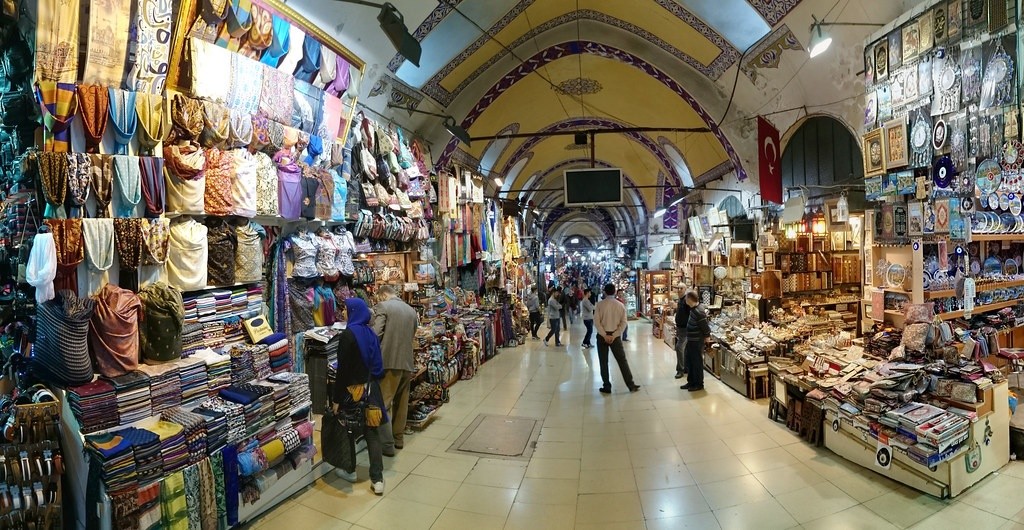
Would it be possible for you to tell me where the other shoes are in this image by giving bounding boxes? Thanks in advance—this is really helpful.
[589,343,594,347]
[395,445,404,450]
[688,385,704,392]
[675,372,684,379]
[555,342,563,346]
[381,453,395,457]
[680,383,690,389]
[599,388,611,393]
[543,338,548,346]
[683,368,688,373]
[581,343,588,348]
[629,385,640,392]
[622,338,630,342]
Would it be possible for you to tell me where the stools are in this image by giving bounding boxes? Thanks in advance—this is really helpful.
[996,348,1024,390]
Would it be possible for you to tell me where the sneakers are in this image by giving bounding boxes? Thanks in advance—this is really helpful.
[371,480,384,493]
[335,467,357,481]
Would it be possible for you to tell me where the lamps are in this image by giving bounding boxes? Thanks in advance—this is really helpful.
[343,1,421,71]
[388,103,471,148]
[808,21,832,58]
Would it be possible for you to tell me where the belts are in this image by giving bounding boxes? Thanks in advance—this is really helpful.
[0,405,67,530]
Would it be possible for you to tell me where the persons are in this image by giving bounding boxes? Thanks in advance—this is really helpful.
[526,286,542,340]
[680,291,711,392]
[543,261,630,348]
[289,225,357,278]
[288,278,353,333]
[593,284,641,393]
[370,285,419,457]
[332,298,389,494]
[675,282,691,378]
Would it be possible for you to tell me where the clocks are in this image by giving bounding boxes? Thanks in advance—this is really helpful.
[853,0,1024,217]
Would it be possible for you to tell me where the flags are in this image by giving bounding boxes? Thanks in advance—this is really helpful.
[758,115,782,205]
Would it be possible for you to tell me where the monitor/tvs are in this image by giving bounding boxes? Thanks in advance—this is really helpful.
[563,167,623,207]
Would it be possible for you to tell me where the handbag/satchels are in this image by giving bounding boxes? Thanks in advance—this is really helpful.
[334,399,363,434]
[354,119,437,219]
[17,263,30,283]
[320,413,356,474]
